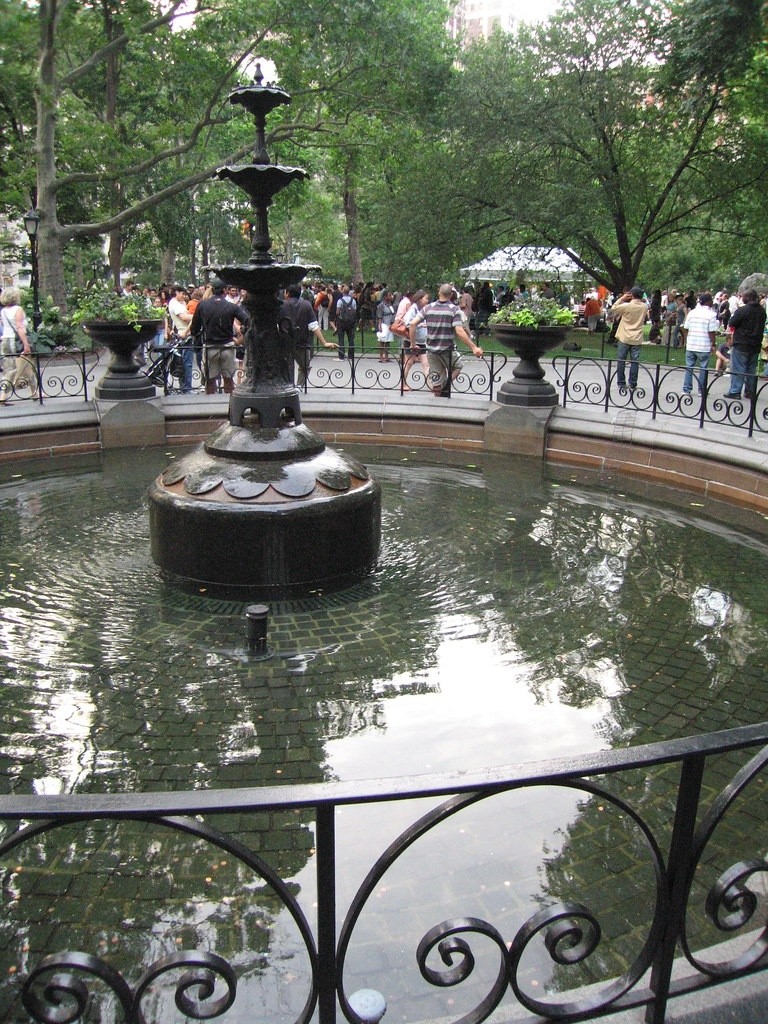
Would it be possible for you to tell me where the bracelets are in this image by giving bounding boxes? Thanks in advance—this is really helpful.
[712,344,716,346]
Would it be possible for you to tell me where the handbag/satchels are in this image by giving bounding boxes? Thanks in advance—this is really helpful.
[389,307,417,340]
[15,332,25,353]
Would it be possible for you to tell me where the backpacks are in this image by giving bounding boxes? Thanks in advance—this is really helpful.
[339,297,356,329]
[320,292,329,308]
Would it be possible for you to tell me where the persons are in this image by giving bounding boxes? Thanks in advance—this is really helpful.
[0,286,45,406]
[122,277,401,394]
[724,288,767,399]
[610,286,649,392]
[642,289,768,348]
[714,334,734,378]
[680,293,717,398]
[581,286,624,345]
[395,281,554,398]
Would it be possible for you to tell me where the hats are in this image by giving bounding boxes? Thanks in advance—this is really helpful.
[187,283,195,288]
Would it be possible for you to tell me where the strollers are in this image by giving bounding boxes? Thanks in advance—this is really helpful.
[144,334,192,388]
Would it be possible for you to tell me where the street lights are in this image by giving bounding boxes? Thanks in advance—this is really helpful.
[91,262,97,276]
[23,210,43,333]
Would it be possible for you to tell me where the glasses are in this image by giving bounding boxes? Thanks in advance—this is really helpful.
[188,287,194,289]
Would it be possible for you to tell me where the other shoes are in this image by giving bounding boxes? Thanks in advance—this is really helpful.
[618,384,628,390]
[745,392,751,400]
[332,358,345,362]
[629,384,636,391]
[697,390,702,397]
[333,329,338,336]
[681,391,691,396]
[370,327,376,332]
[723,392,741,400]
[181,389,200,394]
[33,392,46,400]
[355,327,360,332]
[0,401,15,407]
[378,358,391,363]
[714,371,719,376]
